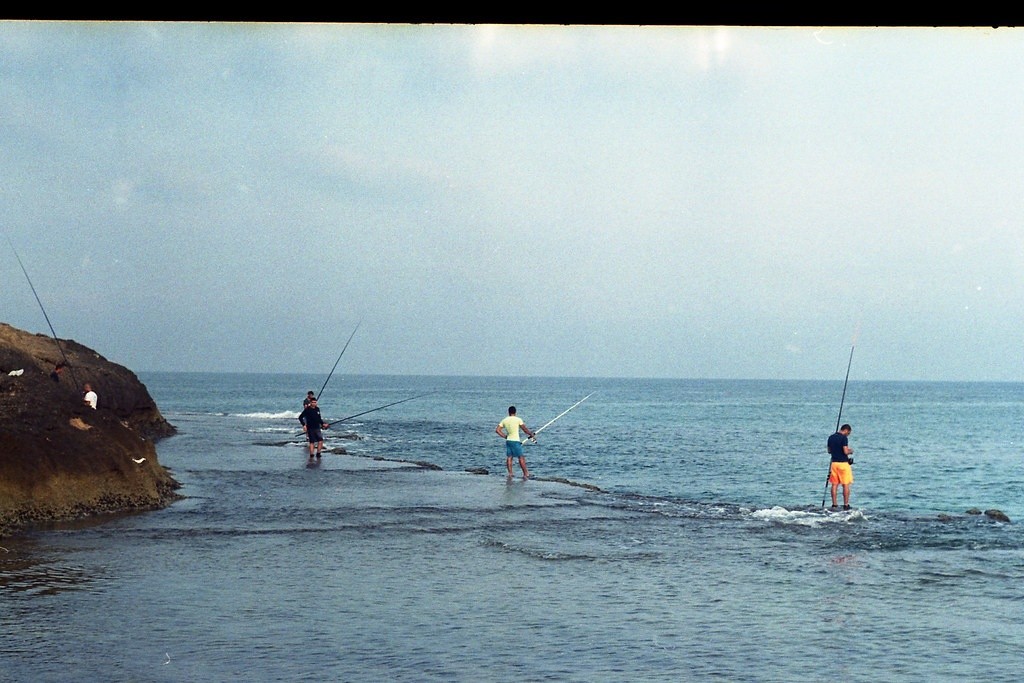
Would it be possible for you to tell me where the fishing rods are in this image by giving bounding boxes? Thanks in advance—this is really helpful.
[817,344,864,515]
[6,239,94,395]
[291,324,437,443]
[520,392,597,447]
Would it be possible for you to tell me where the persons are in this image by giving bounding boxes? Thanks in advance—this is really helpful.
[81,382,98,411]
[298,391,330,459]
[49,363,65,384]
[495,406,535,480]
[827,424,857,511]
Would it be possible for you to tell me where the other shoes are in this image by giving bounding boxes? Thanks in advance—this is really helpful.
[843,504,852,510]
[832,505,839,510]
[315,452,322,458]
[309,453,315,459]
